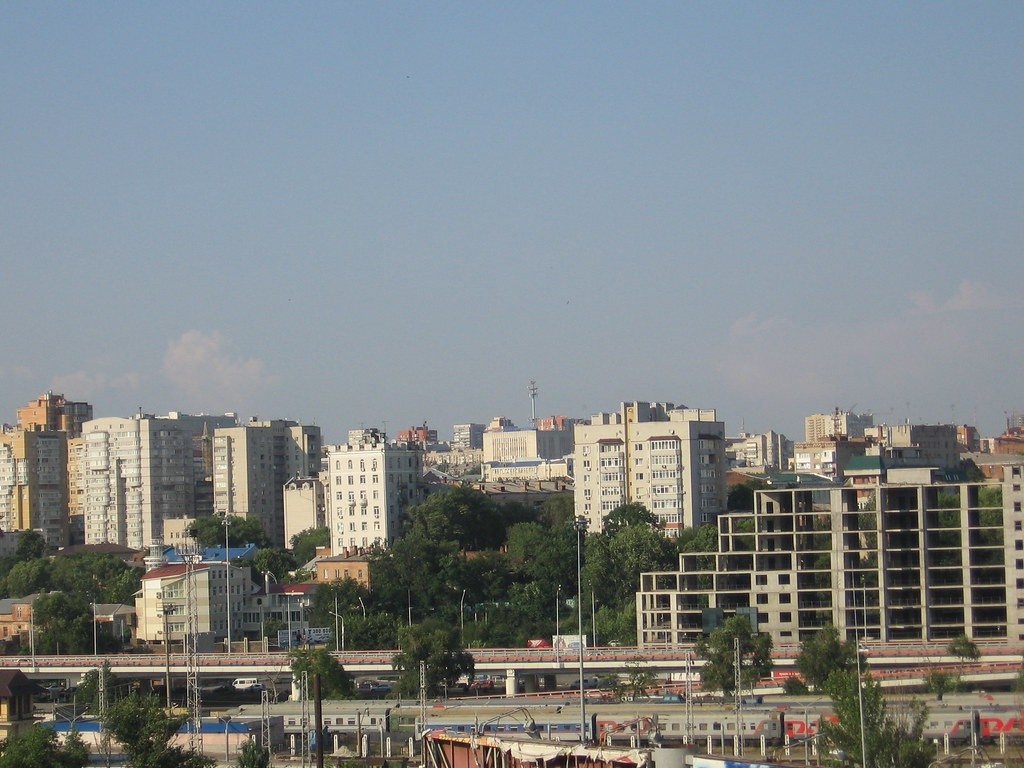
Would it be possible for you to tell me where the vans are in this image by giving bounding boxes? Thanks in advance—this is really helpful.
[232,678,258,689]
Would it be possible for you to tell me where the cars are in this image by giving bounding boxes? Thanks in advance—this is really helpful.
[371,683,393,693]
[470,675,491,690]
[570,677,597,689]
[660,694,686,704]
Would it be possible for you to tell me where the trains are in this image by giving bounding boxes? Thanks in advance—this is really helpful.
[416,704,1024,746]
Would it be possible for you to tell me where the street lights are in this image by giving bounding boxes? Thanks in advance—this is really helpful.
[573,516,590,743]
[329,611,344,650]
[90,603,97,656]
[461,589,467,646]
[222,517,232,655]
